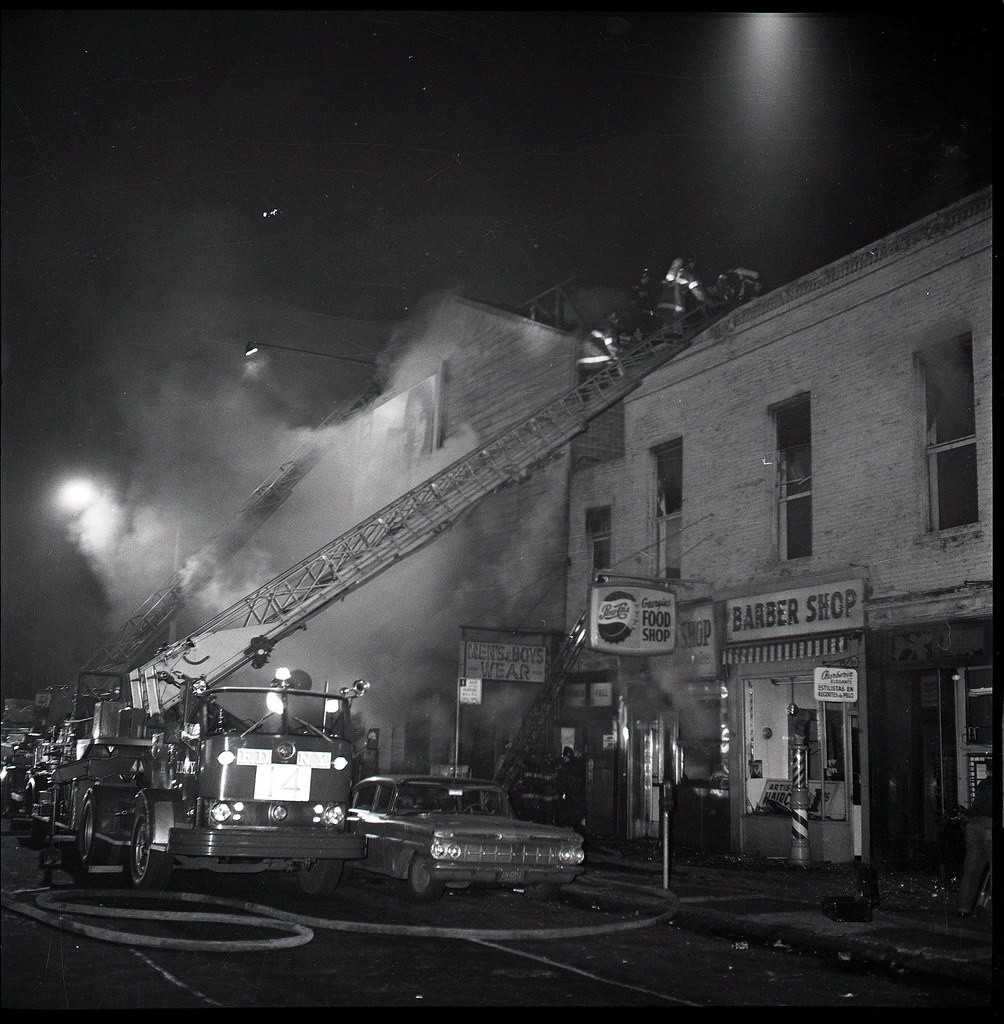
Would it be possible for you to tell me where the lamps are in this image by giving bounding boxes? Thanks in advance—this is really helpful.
[773,681,814,685]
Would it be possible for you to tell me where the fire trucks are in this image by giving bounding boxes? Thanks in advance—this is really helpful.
[0,263,765,897]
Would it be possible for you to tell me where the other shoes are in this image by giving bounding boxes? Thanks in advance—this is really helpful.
[947,901,972,919]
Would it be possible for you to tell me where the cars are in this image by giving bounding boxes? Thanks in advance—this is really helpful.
[341,774,586,905]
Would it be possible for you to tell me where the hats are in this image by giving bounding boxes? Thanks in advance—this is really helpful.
[561,747,574,757]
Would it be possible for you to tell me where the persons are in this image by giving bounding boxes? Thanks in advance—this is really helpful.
[959,759,992,918]
[553,747,586,833]
